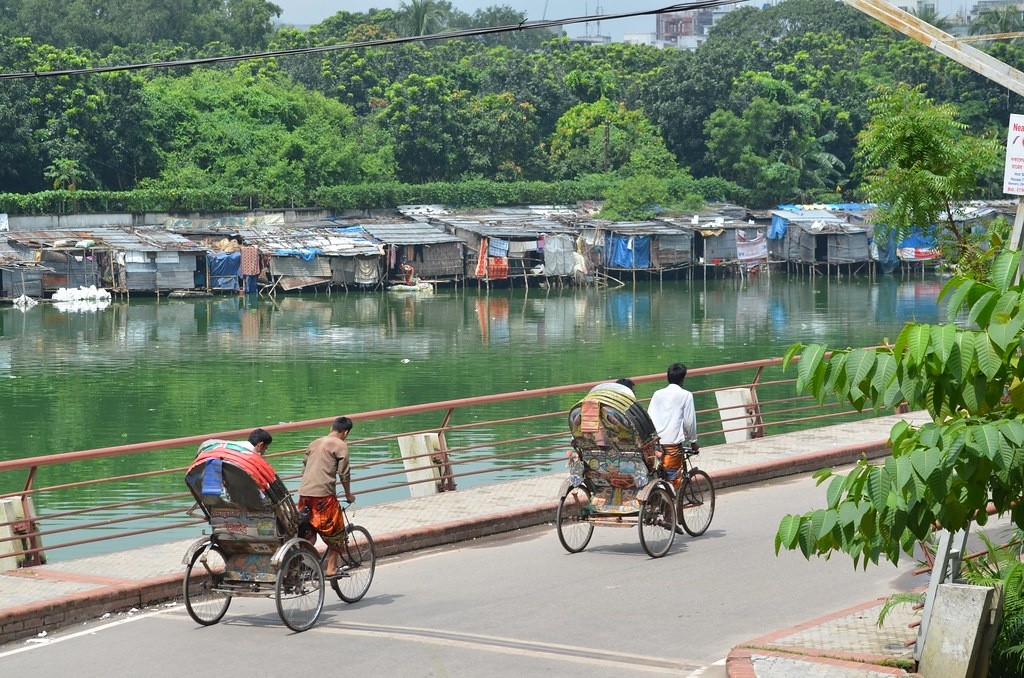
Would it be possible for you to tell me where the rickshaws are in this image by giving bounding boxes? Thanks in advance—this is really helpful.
[556,383,717,558]
[183,439,377,633]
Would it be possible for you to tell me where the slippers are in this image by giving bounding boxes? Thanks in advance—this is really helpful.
[661,522,684,536]
[324,567,351,580]
[643,512,663,526]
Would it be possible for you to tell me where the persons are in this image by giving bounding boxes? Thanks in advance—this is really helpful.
[248,428,273,456]
[298,417,357,580]
[646,363,699,535]
[398,263,414,286]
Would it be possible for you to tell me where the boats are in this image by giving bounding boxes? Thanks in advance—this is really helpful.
[388,282,435,291]
